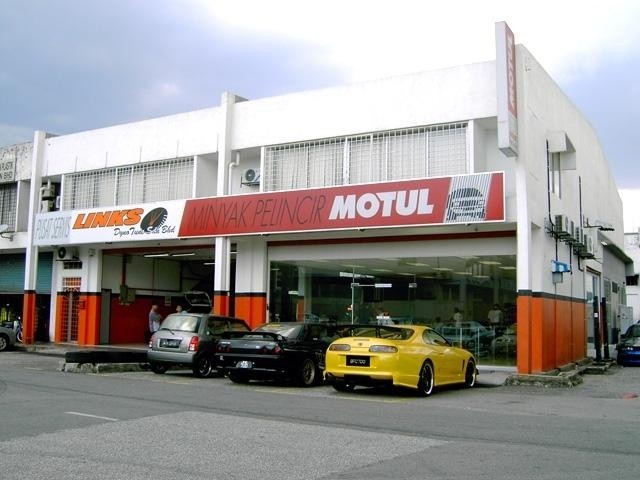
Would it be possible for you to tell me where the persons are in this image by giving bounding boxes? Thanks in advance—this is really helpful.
[451,306,463,328]
[431,316,444,331]
[486,304,505,327]
[175,304,188,313]
[148,301,162,336]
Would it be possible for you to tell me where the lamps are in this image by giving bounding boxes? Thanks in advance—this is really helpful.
[143,252,196,258]
[583,216,615,232]
[0,224,13,241]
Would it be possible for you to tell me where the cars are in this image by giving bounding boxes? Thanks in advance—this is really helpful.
[322,323,480,398]
[0,326,16,351]
[147,313,251,378]
[439,321,495,350]
[618,324,640,366]
[490,323,517,357]
[213,321,344,388]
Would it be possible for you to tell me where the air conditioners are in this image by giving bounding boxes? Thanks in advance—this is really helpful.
[554,214,594,257]
[241,168,261,186]
[55,247,74,261]
[40,184,60,212]
